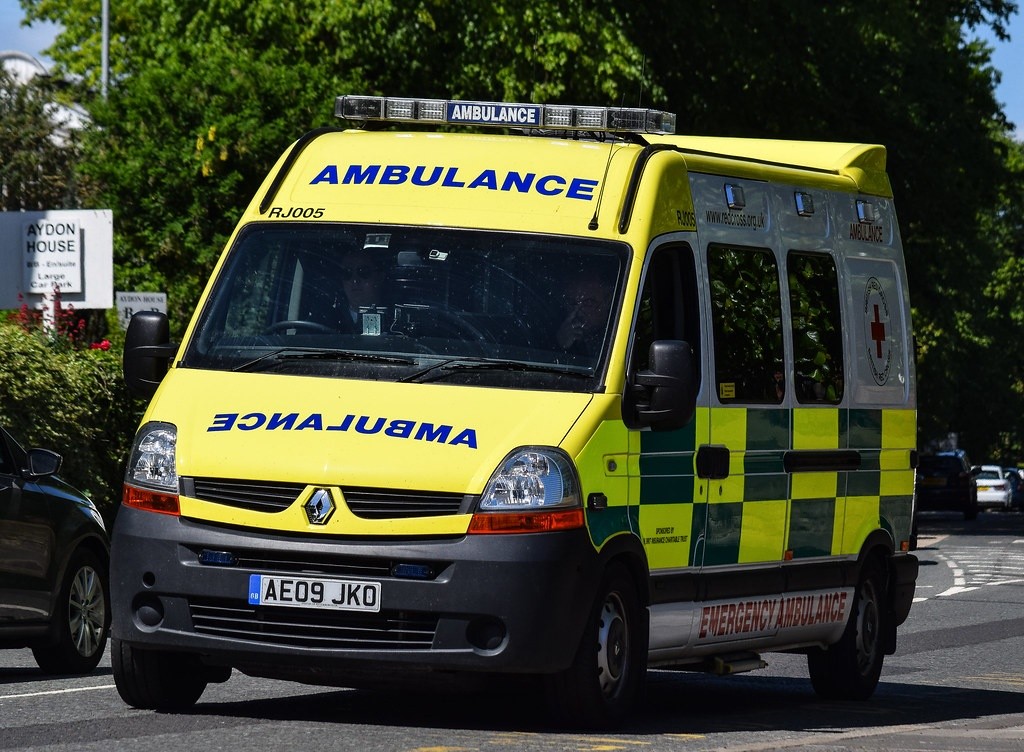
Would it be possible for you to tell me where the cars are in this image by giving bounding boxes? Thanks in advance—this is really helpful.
[972,465,1012,512]
[1004,468,1024,502]
[0,426,113,675]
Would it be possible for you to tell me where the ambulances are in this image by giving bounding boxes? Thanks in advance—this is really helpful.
[113,95,917,713]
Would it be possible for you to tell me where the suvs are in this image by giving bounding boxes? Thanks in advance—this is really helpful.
[917,449,983,521]
